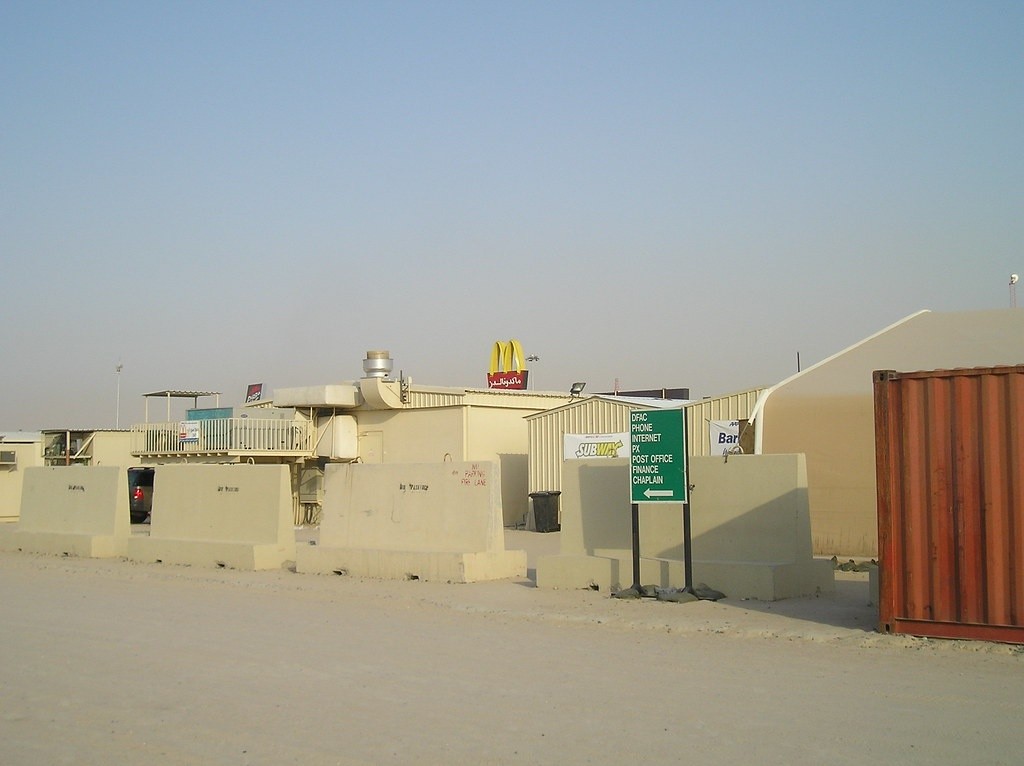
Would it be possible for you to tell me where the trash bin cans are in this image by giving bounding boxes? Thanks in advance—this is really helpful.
[527,491,562,533]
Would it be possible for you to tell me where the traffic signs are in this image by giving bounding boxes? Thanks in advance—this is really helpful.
[628,408,687,505]
